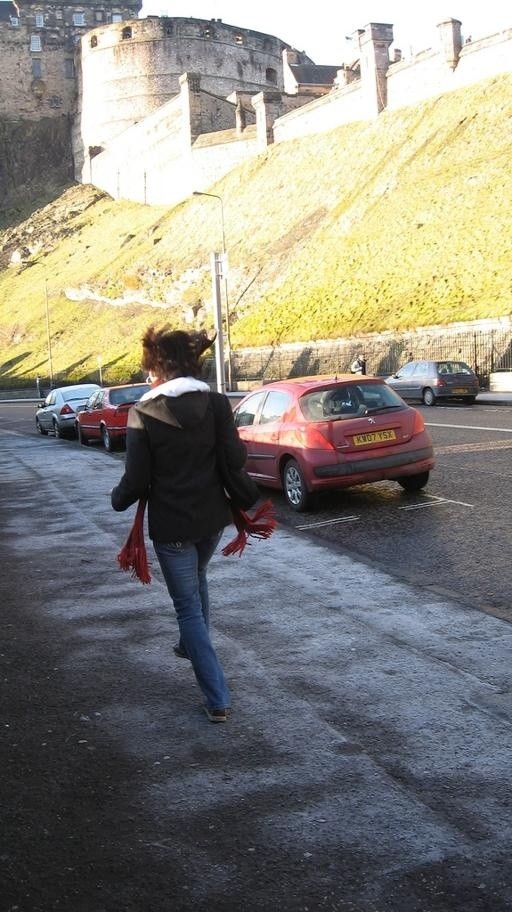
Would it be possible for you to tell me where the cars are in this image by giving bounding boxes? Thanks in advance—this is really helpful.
[76,381,152,452]
[216,371,437,512]
[32,382,101,439]
[384,359,479,407]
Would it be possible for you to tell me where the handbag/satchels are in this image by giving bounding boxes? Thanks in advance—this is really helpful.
[219,435,260,510]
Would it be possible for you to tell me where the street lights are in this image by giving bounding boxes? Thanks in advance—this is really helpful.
[21,258,57,388]
[192,190,236,389]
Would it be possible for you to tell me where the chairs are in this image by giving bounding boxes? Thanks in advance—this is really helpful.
[439,367,447,373]
[115,392,146,402]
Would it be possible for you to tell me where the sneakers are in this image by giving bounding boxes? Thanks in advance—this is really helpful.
[202,704,226,722]
[173,643,192,660]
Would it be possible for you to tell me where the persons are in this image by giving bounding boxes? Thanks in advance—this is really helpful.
[110,322,277,723]
[351,352,365,374]
